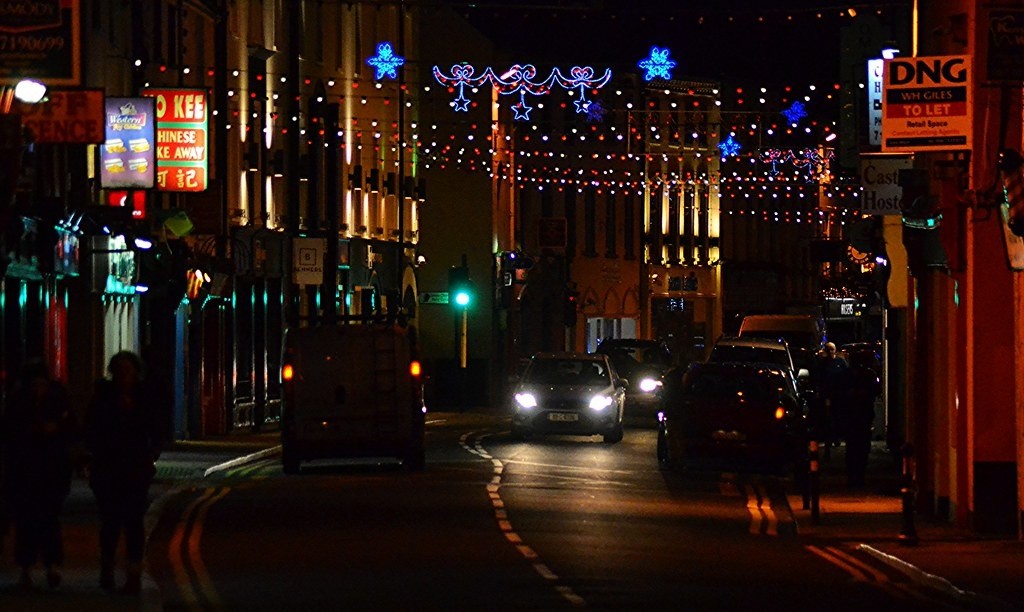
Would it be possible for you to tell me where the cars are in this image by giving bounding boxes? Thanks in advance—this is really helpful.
[706,340,810,380]
[838,342,882,391]
[506,353,630,442]
[658,362,808,479]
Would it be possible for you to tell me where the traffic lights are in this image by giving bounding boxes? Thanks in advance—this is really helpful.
[449,269,472,313]
[566,292,577,314]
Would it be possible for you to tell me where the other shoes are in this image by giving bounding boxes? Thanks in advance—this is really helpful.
[113,578,141,597]
[96,556,117,588]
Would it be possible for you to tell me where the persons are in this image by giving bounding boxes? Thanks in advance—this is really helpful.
[814,342,847,458]
[0,357,79,596]
[86,351,166,600]
[840,351,881,493]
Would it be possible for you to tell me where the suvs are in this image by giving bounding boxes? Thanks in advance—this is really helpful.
[595,336,675,425]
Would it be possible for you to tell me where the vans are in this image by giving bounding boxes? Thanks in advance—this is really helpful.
[279,320,426,472]
[731,313,827,418]
